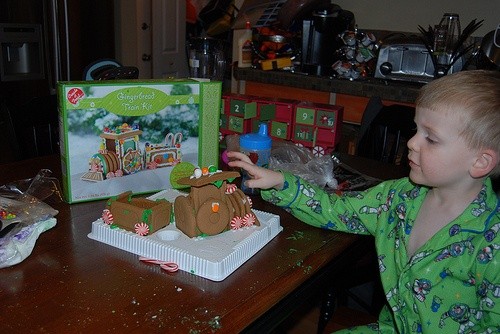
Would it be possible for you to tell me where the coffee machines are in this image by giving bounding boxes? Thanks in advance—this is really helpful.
[301,4,355,76]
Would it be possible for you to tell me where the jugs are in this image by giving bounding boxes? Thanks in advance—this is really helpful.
[187,35,228,93]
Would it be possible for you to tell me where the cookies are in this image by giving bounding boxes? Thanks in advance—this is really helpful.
[80,123,181,182]
[173,166,260,238]
[102,190,174,237]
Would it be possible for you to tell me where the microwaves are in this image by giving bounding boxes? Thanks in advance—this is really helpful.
[0,23,41,82]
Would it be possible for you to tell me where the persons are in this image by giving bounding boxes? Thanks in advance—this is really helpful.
[227,70,500,334]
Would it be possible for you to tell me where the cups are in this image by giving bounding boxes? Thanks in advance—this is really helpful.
[432,12,461,56]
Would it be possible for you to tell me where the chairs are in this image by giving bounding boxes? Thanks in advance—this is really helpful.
[354,106,426,179]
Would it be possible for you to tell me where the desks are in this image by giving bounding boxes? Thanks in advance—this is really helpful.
[234,66,423,125]
[0,154,360,334]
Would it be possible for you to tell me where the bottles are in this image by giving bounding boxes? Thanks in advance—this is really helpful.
[477,27,500,72]
[241,122,272,196]
[238,21,252,68]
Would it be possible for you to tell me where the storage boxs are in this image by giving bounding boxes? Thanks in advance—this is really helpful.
[57,79,222,204]
